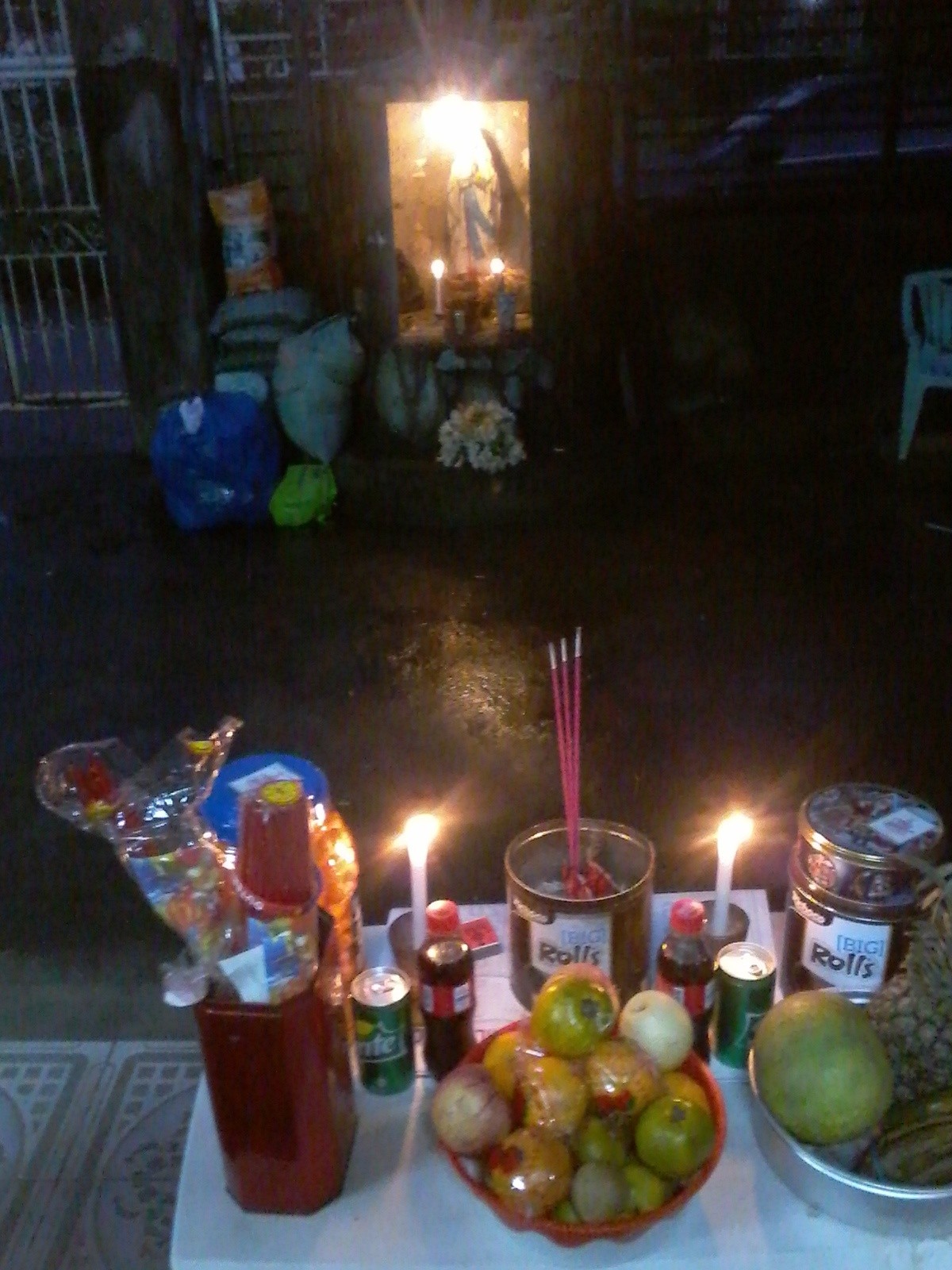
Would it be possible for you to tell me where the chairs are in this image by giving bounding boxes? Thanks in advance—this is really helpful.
[898,267,951,460]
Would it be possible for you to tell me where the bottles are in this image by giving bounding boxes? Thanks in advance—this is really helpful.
[446,277,474,343]
[783,781,944,998]
[417,899,478,1082]
[658,900,713,1064]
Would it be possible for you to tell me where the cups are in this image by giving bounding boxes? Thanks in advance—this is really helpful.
[197,905,358,1216]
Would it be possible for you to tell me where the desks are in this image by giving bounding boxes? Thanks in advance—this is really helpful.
[169,890,952,1270]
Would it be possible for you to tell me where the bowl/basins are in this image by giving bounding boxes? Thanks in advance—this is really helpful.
[438,1016,726,1247]
[748,987,952,1235]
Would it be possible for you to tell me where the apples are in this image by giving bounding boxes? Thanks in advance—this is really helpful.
[538,964,714,1224]
[431,1064,508,1155]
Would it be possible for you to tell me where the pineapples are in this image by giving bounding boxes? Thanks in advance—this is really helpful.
[860,846,952,1104]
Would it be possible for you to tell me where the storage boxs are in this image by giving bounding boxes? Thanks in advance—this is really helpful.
[460,917,503,961]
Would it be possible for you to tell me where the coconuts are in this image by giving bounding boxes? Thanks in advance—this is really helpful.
[751,991,893,1147]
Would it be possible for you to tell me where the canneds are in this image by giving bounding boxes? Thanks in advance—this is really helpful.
[780,783,947,1013]
[349,965,416,1094]
[708,942,776,1068]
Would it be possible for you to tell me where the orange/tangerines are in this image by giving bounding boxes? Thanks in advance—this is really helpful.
[476,1032,714,1219]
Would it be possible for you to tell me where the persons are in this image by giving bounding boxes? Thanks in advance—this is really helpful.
[446,132,502,276]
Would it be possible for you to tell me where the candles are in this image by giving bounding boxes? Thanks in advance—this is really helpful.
[432,258,443,314]
[404,811,441,950]
[709,813,753,935]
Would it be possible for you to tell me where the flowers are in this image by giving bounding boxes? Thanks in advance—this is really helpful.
[435,399,527,473]
